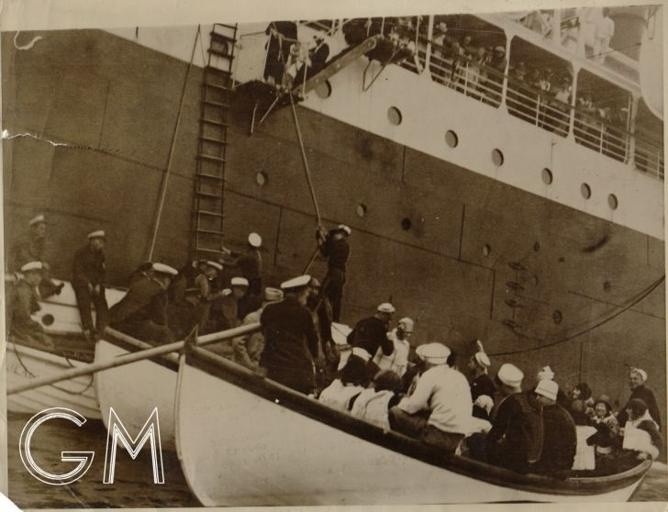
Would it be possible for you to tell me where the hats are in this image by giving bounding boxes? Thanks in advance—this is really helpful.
[28,215,48,227]
[475,351,648,420]
[397,317,414,333]
[247,232,262,247]
[280,275,312,293]
[423,341,451,363]
[338,224,351,235]
[221,288,232,295]
[264,287,283,301]
[207,260,223,271]
[21,261,43,273]
[309,277,321,288]
[152,262,178,279]
[416,344,426,360]
[87,230,105,240]
[231,276,249,287]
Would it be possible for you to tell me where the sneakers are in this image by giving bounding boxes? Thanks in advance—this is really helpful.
[56,282,64,295]
[32,304,42,313]
[84,327,95,340]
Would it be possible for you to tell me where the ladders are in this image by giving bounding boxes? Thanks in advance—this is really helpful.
[194,23,239,288]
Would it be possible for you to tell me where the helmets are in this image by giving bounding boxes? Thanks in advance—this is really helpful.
[377,302,396,313]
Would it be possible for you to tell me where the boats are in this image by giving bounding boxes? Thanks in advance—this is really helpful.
[1,1,668,462]
